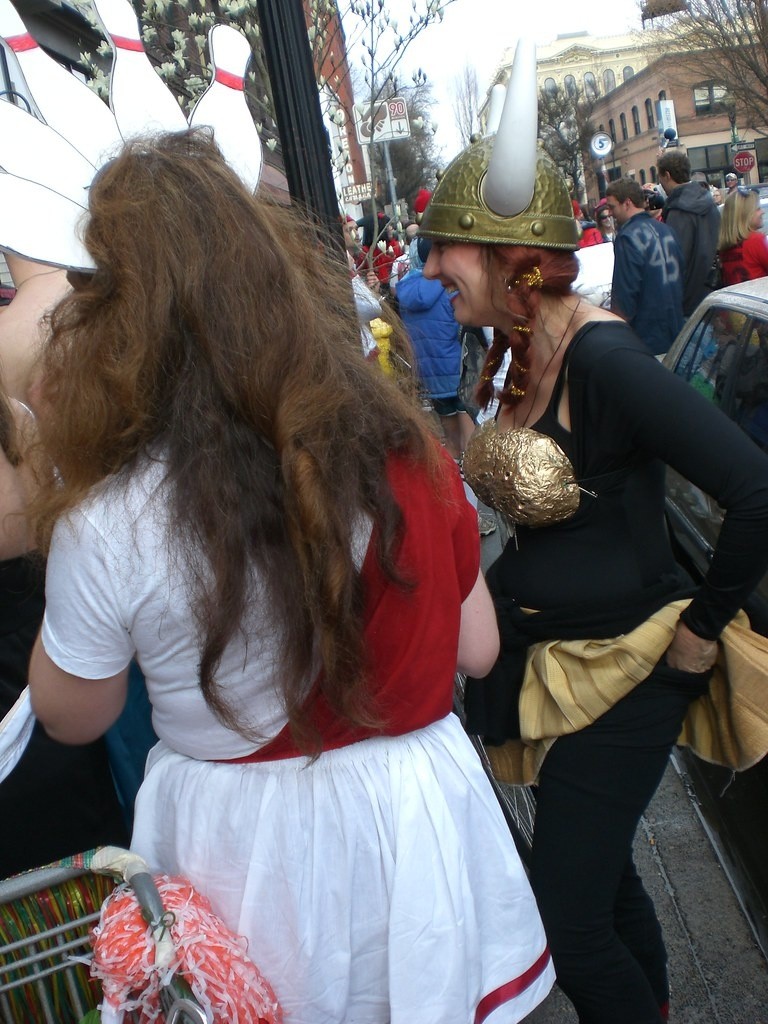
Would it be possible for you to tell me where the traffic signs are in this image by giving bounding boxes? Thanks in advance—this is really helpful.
[731,142,756,152]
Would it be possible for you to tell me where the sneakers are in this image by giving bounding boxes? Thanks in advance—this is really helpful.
[478,514,497,536]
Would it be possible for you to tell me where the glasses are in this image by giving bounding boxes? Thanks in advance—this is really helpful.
[598,214,613,221]
[738,186,759,197]
[726,178,736,181]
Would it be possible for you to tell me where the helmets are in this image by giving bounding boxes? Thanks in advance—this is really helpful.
[415,34,581,250]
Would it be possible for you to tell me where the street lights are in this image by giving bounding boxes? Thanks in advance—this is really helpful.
[721,93,737,143]
[610,142,617,180]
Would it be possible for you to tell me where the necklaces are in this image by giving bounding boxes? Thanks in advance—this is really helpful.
[513,299,583,430]
[15,268,60,294]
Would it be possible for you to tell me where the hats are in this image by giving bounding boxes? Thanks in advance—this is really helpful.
[691,173,710,191]
[418,235,432,264]
[726,173,738,181]
[340,214,355,226]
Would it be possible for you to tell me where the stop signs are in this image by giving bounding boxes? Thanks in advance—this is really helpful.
[733,151,755,173]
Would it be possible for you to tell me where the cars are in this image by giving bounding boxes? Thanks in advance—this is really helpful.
[717,198,768,240]
[659,278,767,638]
[737,183,768,198]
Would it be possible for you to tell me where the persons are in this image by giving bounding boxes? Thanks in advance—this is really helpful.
[1,134,768,1024]
